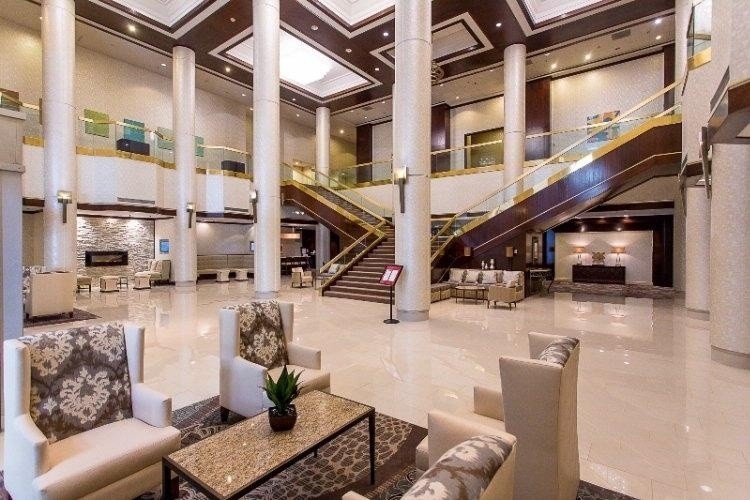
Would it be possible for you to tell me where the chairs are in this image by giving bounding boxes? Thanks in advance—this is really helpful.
[413,327,583,499]
[340,409,520,500]
[24,252,172,327]
[217,300,330,423]
[1,320,183,500]
[431,265,528,309]
[291,263,349,290]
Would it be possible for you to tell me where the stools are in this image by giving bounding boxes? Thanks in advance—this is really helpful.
[234,269,250,280]
[216,268,230,283]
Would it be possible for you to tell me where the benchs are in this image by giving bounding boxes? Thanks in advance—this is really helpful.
[197,253,255,279]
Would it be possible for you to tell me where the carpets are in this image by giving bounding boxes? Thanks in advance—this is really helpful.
[543,277,678,300]
[0,391,644,499]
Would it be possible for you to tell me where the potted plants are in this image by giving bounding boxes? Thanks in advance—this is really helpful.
[256,367,309,431]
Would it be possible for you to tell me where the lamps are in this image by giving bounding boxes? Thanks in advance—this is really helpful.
[463,245,472,266]
[56,188,74,223]
[393,166,408,214]
[505,247,514,270]
[248,189,259,223]
[611,244,627,268]
[572,247,586,264]
[186,201,197,230]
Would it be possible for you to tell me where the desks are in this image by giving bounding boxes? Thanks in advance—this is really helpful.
[161,388,378,500]
[574,264,628,287]
[526,266,555,295]
